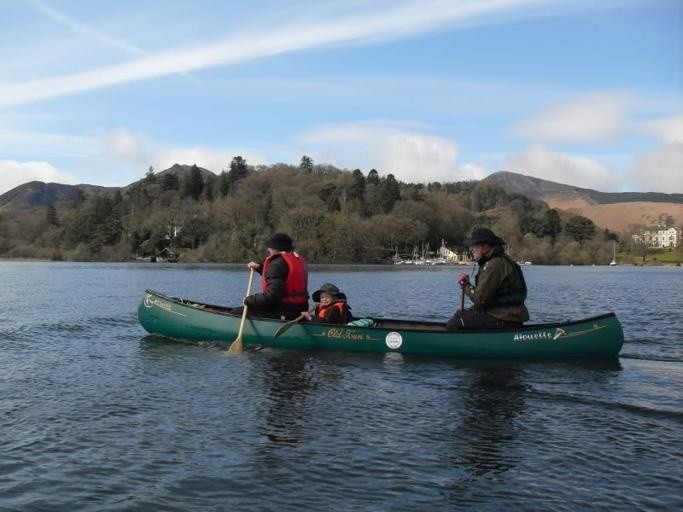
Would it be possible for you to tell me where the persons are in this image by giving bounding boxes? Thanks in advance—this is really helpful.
[446,226,530,333]
[228,233,309,320]
[301,284,354,324]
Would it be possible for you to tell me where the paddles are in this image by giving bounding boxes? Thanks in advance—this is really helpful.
[272,306,315,340]
[227,266,254,353]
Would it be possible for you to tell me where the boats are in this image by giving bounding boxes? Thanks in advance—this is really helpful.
[136,290,625,366]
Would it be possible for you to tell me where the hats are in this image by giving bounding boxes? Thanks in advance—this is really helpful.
[266,233,295,252]
[461,227,505,246]
[312,284,346,302]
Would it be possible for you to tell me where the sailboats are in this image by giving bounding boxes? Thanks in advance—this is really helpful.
[390,238,532,266]
[608,239,617,265]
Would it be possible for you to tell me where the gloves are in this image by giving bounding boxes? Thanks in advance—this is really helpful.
[458,272,469,286]
